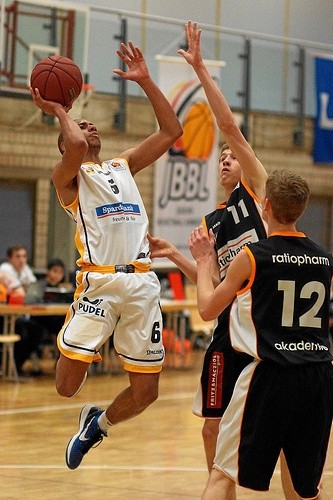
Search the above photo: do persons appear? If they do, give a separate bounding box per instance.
[188,171,333,500]
[147,19,268,500]
[0,243,42,376]
[25,258,74,379]
[27,40,183,469]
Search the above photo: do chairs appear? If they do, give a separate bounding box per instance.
[186,286,216,370]
[0,332,20,382]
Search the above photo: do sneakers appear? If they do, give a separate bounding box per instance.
[65,403,108,469]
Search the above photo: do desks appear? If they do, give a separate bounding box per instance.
[0,299,199,384]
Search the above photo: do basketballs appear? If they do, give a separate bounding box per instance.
[30,55,84,108]
